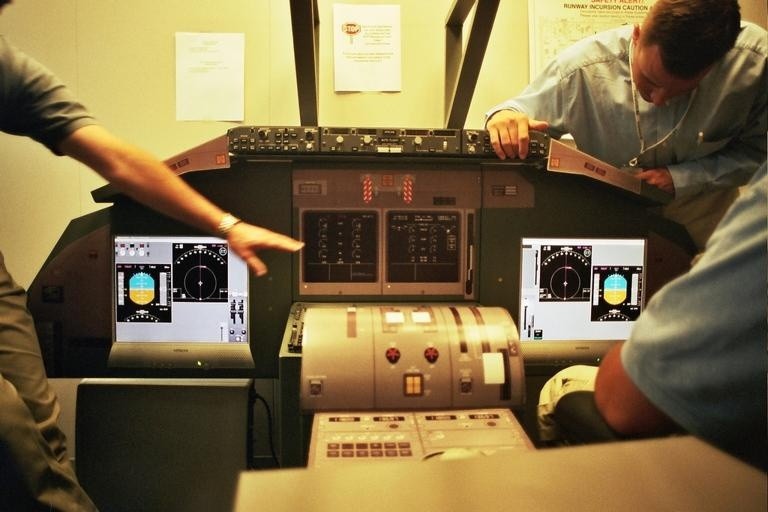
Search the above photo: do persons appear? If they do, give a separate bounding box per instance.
[532,158,766,485]
[0,0,308,512]
[480,0,766,248]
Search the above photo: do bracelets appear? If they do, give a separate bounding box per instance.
[215,214,240,239]
